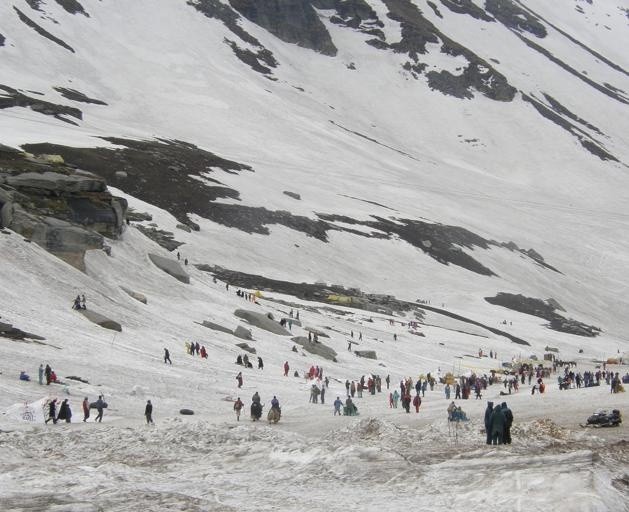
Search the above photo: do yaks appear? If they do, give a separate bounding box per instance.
[267,406,282,425]
[251,402,265,422]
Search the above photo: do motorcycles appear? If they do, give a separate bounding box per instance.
[578,408,623,432]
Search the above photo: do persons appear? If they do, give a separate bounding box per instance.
[176,245,629,446]
[20,370,30,381]
[145,400,153,424]
[81,294,88,308]
[164,348,172,365]
[38,362,108,425]
[75,295,81,310]
